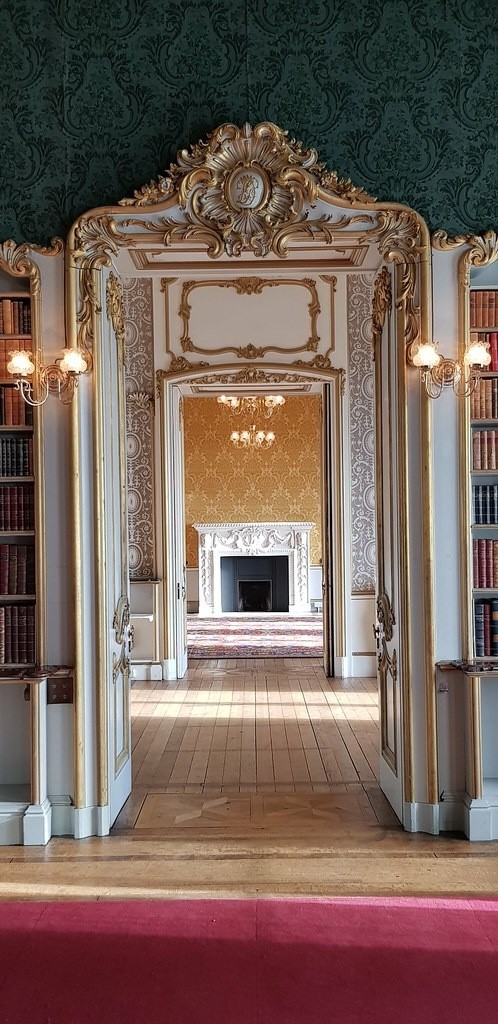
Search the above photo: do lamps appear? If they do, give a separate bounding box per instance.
[412,341,492,399]
[216,395,287,447]
[8,347,89,408]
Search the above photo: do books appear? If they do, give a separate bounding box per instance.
[466,292,497,659]
[2,297,36,670]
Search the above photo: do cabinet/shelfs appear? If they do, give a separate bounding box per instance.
[0,294,44,678]
[463,287,498,661]
